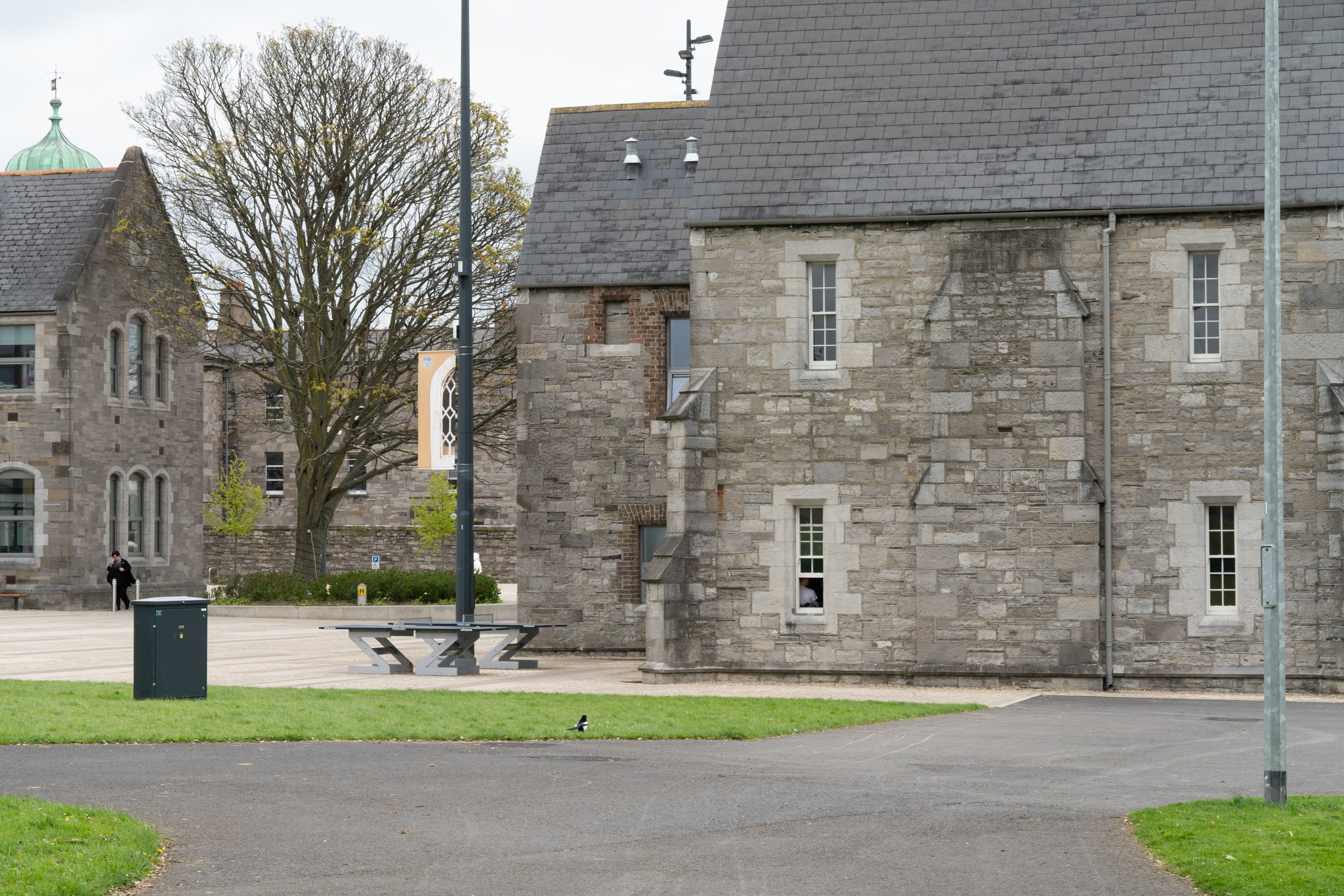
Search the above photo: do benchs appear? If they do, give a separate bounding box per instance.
[0,593,29,610]
[318,614,568,676]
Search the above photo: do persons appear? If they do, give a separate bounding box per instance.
[799,577,818,605]
[107,551,136,611]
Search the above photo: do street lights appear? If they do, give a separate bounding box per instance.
[664,18,714,100]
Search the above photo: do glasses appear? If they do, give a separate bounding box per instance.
[112,556,117,558]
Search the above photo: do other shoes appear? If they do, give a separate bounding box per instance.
[125,602,130,610]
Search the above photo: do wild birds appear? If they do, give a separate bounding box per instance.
[565,714,589,733]
